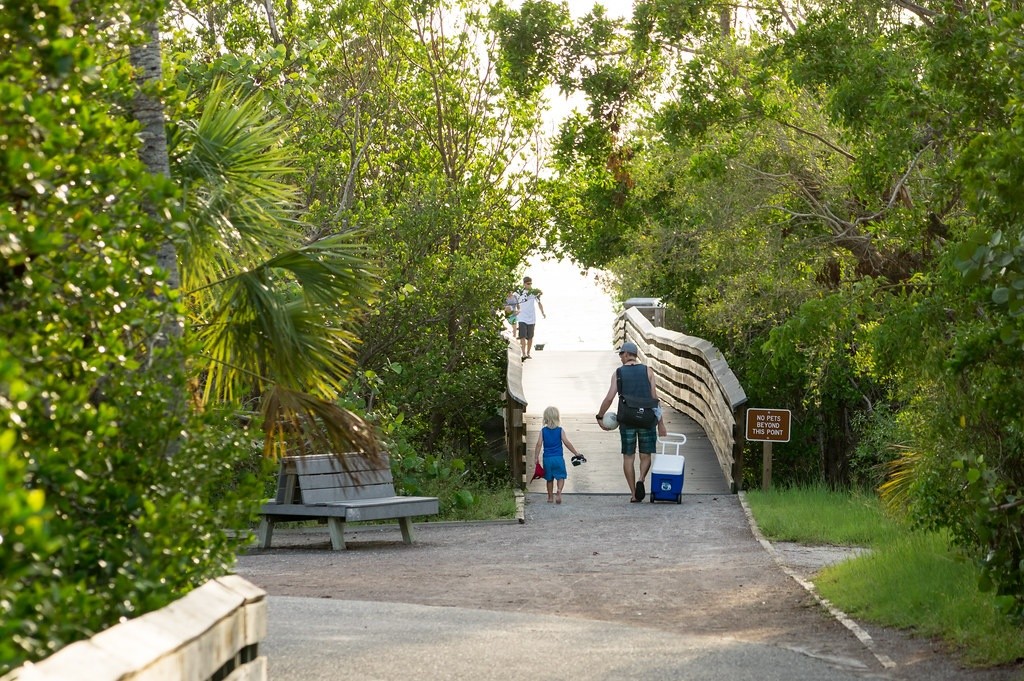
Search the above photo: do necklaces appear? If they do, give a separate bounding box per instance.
[626,359,636,362]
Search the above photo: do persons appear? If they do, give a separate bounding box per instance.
[534,406,580,503]
[506,292,521,337]
[597,342,667,502]
[518,277,546,361]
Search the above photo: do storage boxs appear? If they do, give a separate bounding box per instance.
[650,433,687,504]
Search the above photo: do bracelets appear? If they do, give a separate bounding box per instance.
[596,415,603,420]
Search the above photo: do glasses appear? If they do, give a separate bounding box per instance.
[526,282,532,285]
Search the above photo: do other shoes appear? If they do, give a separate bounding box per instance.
[571,454,587,466]
[522,355,528,362]
[527,355,532,359]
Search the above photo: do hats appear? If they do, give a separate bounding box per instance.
[529,463,546,484]
[615,342,637,355]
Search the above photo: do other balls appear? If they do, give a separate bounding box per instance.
[601,412,619,431]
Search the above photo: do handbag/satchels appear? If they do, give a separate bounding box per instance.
[616,394,662,428]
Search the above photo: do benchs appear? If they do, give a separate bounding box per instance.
[250,451,439,552]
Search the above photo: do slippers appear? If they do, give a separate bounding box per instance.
[630,497,641,502]
[634,481,645,501]
